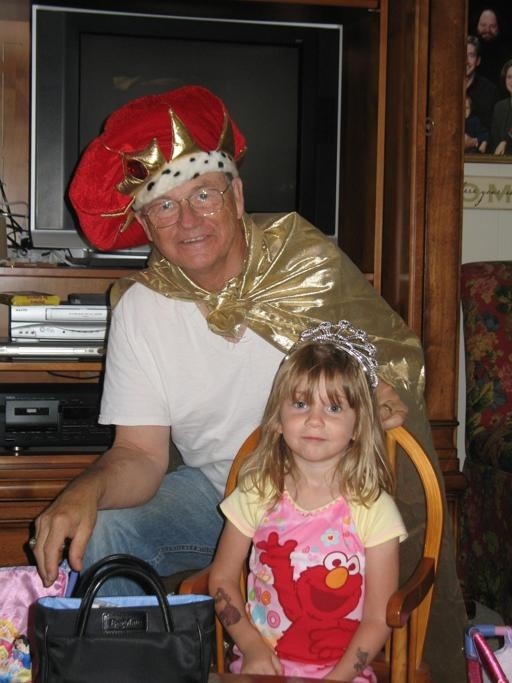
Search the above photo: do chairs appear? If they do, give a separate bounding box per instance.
[179,407,446,683]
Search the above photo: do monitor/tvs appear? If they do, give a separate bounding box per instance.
[30,3,344,266]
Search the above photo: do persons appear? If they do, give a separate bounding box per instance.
[464,9,512,158]
[33,83,470,682]
[207,320,409,683]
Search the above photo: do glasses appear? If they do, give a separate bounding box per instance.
[140,185,229,229]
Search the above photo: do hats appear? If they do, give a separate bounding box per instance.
[68,86,247,252]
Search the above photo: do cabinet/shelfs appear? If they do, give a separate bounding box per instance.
[0,263,147,566]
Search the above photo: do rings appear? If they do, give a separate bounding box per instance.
[384,403,392,415]
[29,539,35,547]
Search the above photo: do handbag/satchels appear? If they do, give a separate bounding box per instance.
[29,554,214,682]
